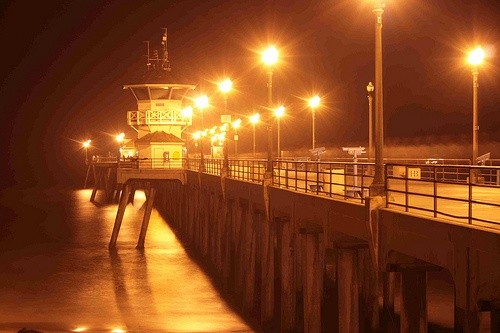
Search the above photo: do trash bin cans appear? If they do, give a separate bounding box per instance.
[322,168,345,199]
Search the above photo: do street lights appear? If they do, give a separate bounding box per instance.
[181,77,244,170]
[83,140,91,178]
[249,112,260,160]
[262,44,285,179]
[115,133,126,164]
[467,45,486,178]
[308,95,321,158]
[366,81,374,179]
